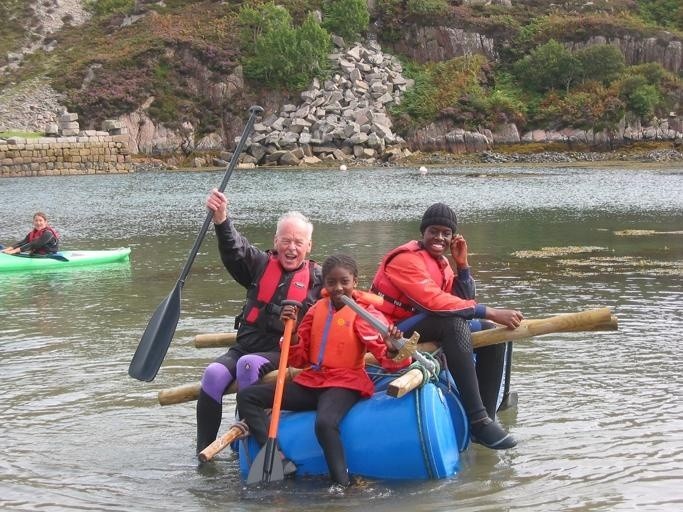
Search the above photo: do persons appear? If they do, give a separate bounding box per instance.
[369,202,524,450]
[235,252,413,495]
[195,190,326,463]
[2,211,59,258]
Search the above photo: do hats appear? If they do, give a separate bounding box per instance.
[421,203,457,232]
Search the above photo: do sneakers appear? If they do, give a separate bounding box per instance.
[470,415,518,450]
[282,458,297,475]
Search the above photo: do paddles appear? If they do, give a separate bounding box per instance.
[128,106,263,382]
[0,243,67,263]
[247,300,301,485]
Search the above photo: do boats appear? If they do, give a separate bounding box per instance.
[0,248,131,272]
